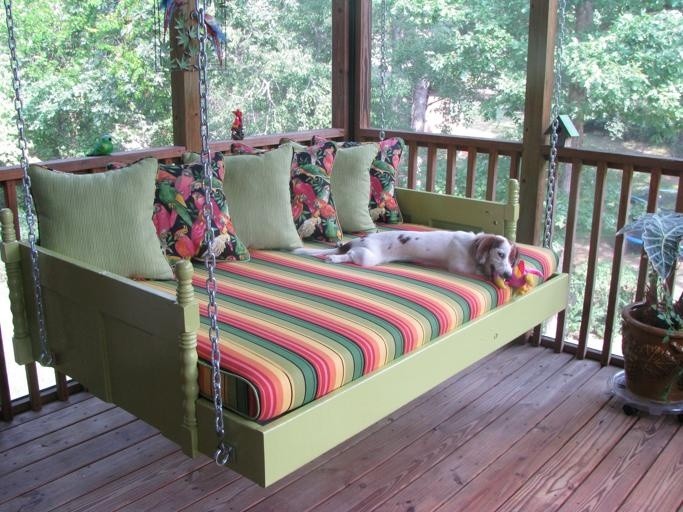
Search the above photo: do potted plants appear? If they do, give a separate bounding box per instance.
[614,209,683,403]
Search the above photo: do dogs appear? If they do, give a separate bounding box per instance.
[291,229,519,282]
